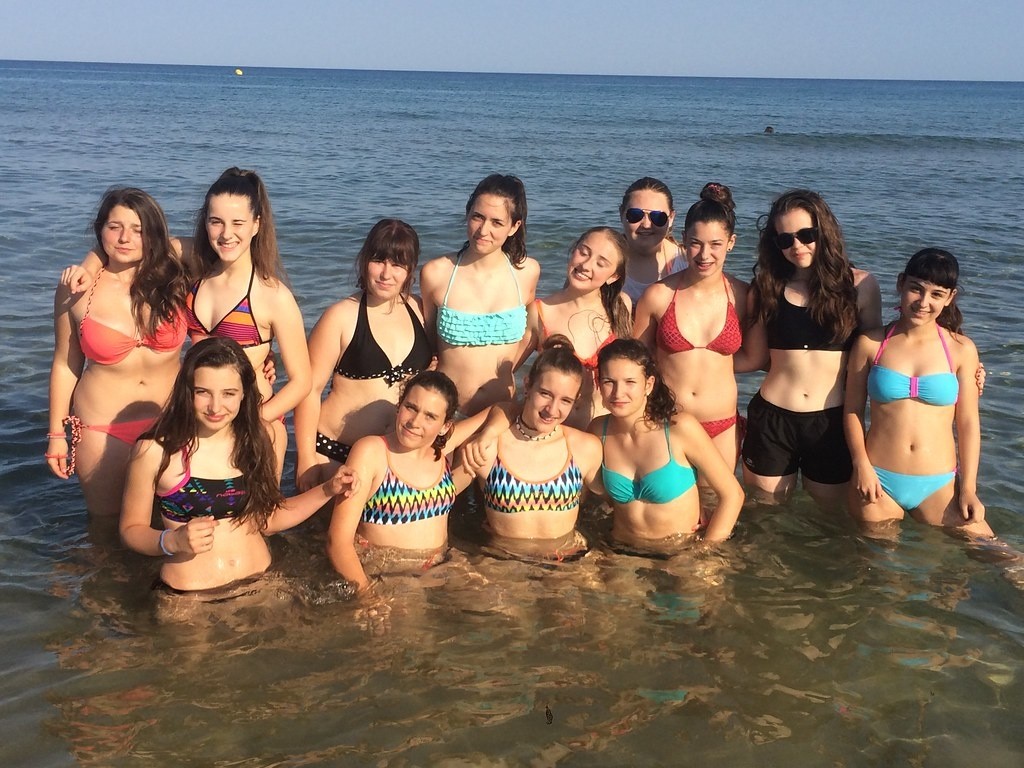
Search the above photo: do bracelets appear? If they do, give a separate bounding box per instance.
[160,529,176,555]
[44,431,69,458]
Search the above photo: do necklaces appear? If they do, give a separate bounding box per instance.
[515,415,561,442]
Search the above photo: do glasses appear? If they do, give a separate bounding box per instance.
[774,226,819,249]
[620,207,671,227]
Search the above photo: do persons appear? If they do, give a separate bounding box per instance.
[327,371,523,590]
[119,336,360,591]
[564,176,689,314]
[634,183,769,486]
[46,182,276,513]
[295,218,437,506]
[511,226,632,505]
[765,126,773,133]
[420,173,541,420]
[60,165,312,497]
[842,247,995,538]
[578,338,745,541]
[733,189,986,508]
[451,333,605,538]
